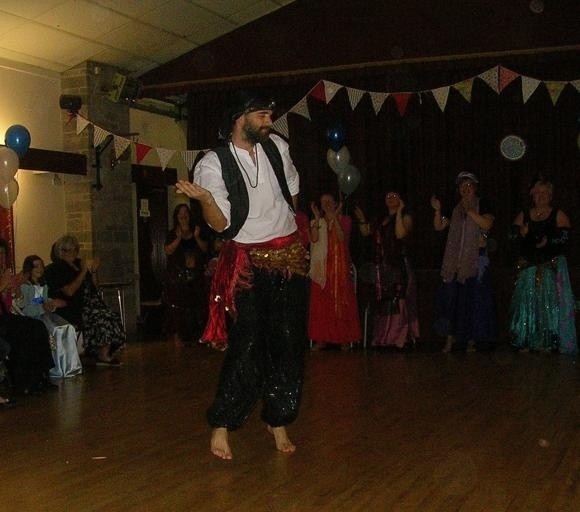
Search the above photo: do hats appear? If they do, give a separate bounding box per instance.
[223,89,276,123]
[458,172,480,184]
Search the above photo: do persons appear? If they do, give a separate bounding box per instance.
[0,392,20,411]
[12,253,87,379]
[41,234,129,367]
[175,88,310,462]
[0,238,60,399]
[176,200,226,353]
[293,172,578,357]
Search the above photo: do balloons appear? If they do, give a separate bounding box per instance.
[155,167,176,186]
[325,119,347,154]
[324,144,350,175]
[3,124,32,163]
[337,163,362,195]
[0,144,20,191]
[1,177,20,211]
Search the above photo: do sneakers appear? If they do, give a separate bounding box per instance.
[94,356,125,367]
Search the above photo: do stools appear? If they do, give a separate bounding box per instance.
[97,282,131,349]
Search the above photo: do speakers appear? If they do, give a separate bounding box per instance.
[59,94,81,111]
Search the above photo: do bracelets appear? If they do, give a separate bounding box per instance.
[90,271,98,274]
